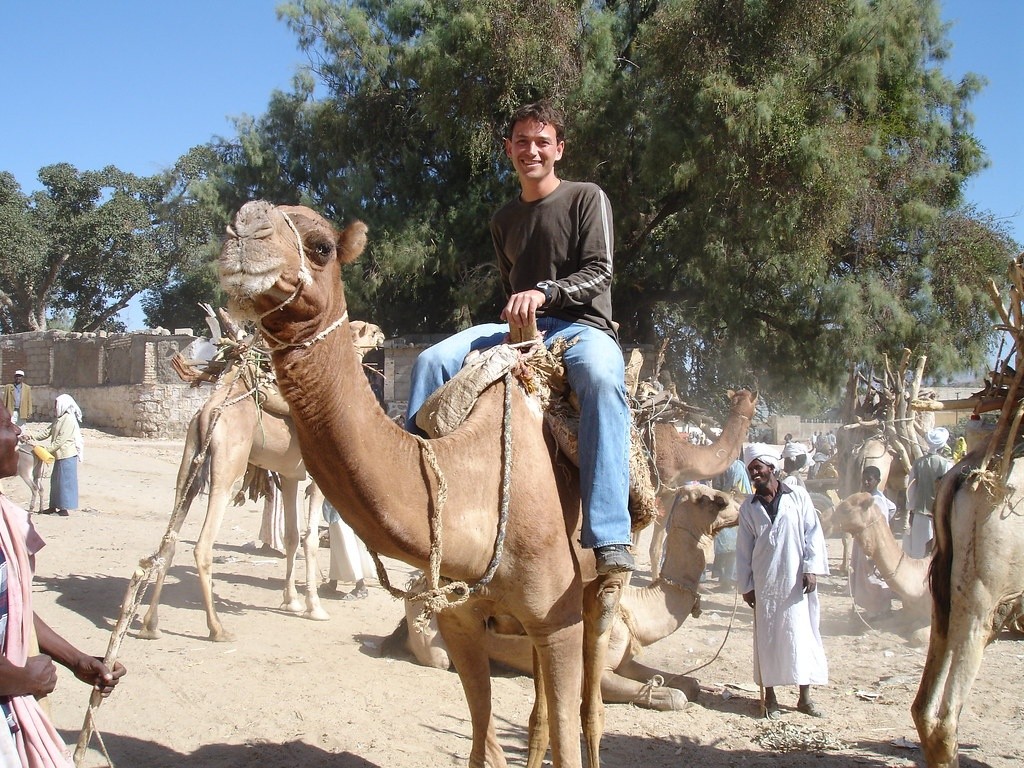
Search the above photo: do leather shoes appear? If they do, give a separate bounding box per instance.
[593,544,635,575]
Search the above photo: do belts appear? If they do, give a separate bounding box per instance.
[14,408,19,411]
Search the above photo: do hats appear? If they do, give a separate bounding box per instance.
[926,427,949,454]
[15,370,24,376]
[745,444,780,469]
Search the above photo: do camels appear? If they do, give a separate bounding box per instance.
[813,392,1024,768]
[216,197,760,768]
[134,318,386,638]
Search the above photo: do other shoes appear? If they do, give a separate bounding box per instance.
[320,583,335,591]
[797,700,821,717]
[43,509,56,514]
[59,510,68,516]
[342,589,369,600]
[765,697,780,720]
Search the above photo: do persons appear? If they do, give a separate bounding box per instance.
[806,430,837,502]
[902,427,949,559]
[710,459,753,589]
[0,399,127,768]
[257,470,305,556]
[3,369,32,430]
[735,442,832,721]
[779,434,793,461]
[844,465,897,620]
[406,105,636,577]
[952,436,968,466]
[318,496,370,603]
[679,430,724,448]
[781,442,806,489]
[19,393,84,516]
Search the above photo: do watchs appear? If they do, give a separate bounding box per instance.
[533,281,553,308]
